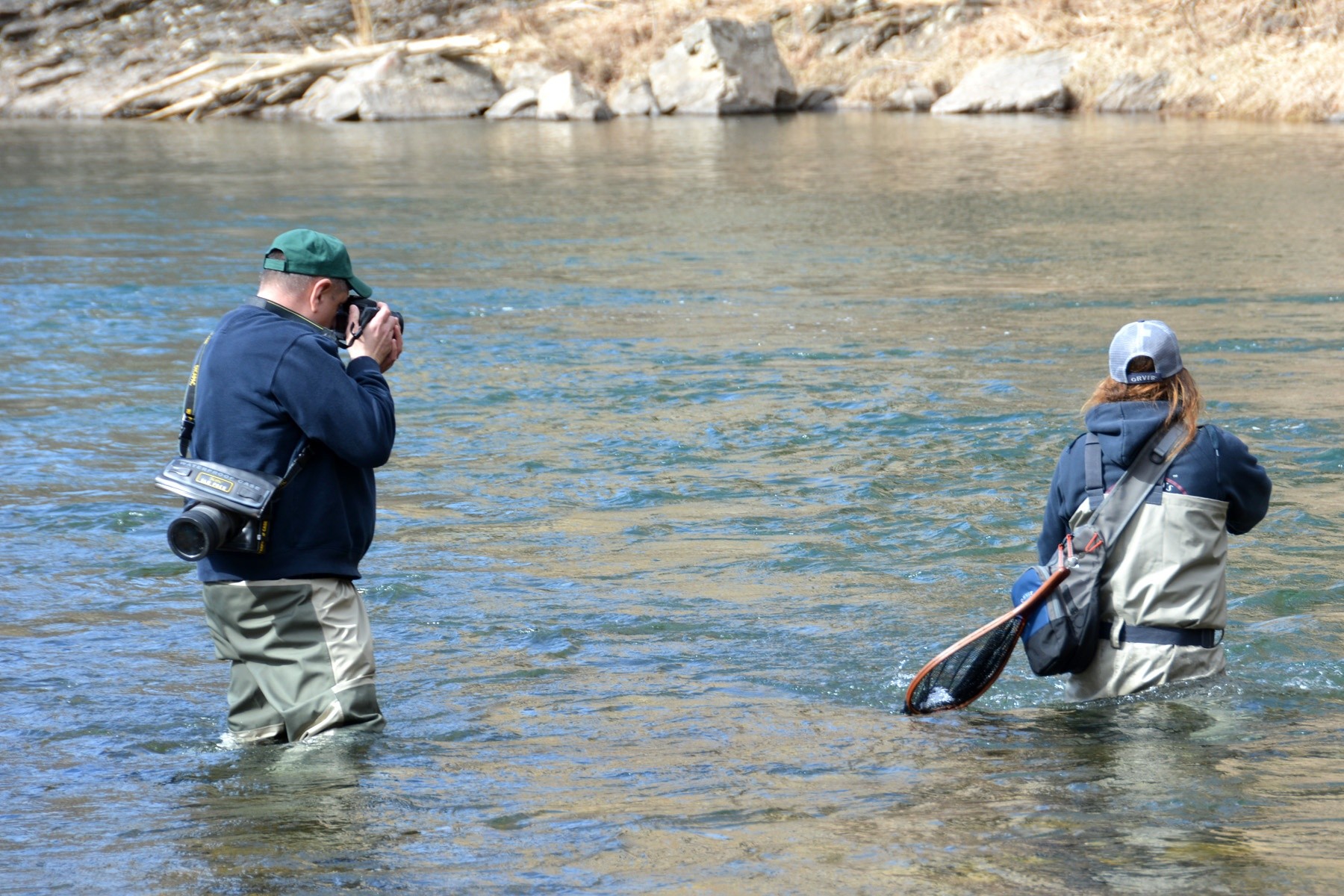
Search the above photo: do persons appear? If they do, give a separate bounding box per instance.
[177,229,403,744]
[1037,321,1272,702]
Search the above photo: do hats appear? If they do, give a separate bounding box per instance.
[263,229,373,298]
[1108,319,1184,384]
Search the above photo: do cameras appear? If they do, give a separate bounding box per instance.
[334,293,405,339]
[167,501,232,562]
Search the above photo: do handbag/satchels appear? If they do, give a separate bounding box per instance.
[1011,526,1107,678]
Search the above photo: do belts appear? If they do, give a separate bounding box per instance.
[1098,620,1215,650]
